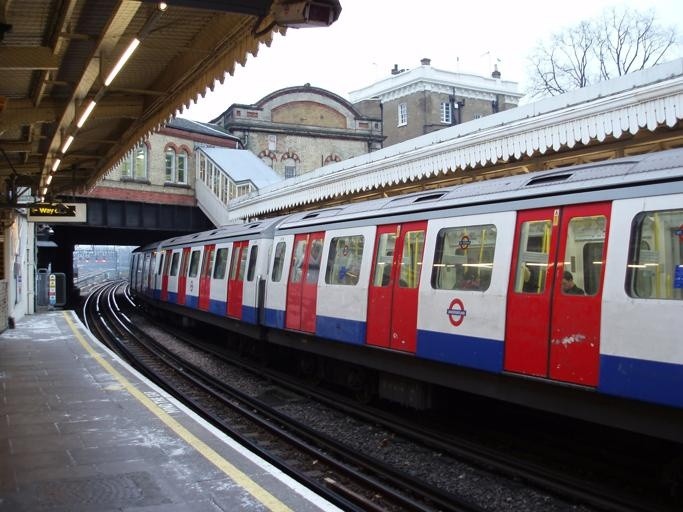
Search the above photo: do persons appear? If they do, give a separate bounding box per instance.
[563,270,586,295]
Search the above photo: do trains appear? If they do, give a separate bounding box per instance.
[127,145,682,446]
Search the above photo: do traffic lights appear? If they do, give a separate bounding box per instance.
[101,256,105,263]
[86,257,88,263]
[95,257,98,263]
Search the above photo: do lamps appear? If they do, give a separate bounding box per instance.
[36,3,170,202]
